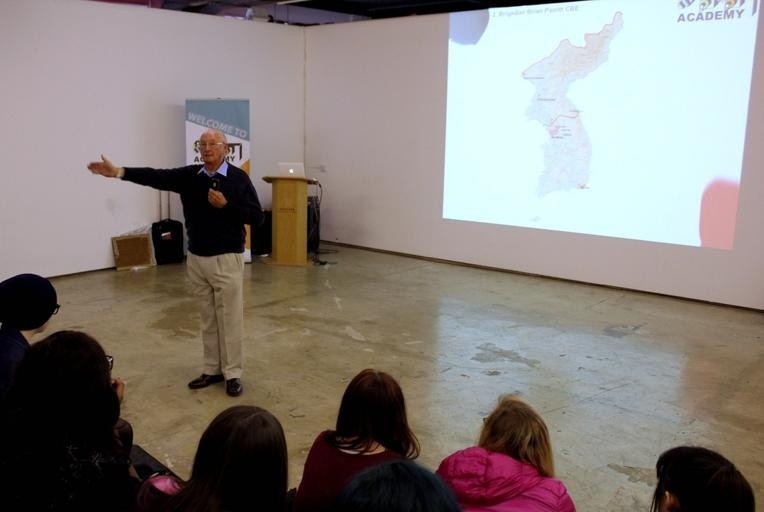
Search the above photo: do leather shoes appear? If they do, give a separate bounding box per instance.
[226,378,243,397]
[188,373,223,389]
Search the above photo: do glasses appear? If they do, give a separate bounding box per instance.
[52,304,60,315]
[106,355,114,371]
[197,143,223,150]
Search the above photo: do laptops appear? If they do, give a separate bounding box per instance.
[278,162,306,177]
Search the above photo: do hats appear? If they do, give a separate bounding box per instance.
[1,274,57,330]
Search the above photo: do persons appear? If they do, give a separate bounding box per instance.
[134,404,297,511]
[1,330,156,511]
[294,366,420,512]
[648,444,756,512]
[87,127,263,398]
[323,458,463,512]
[434,390,579,512]
[1,271,62,388]
